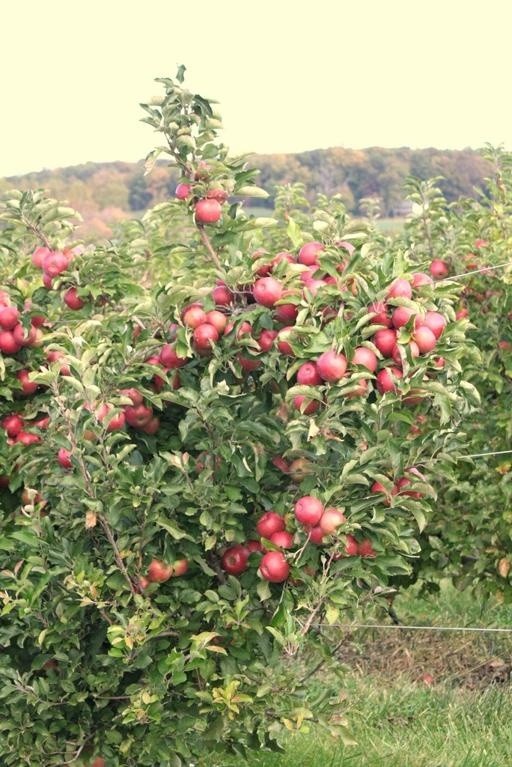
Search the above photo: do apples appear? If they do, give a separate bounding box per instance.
[0,160,512,592]
